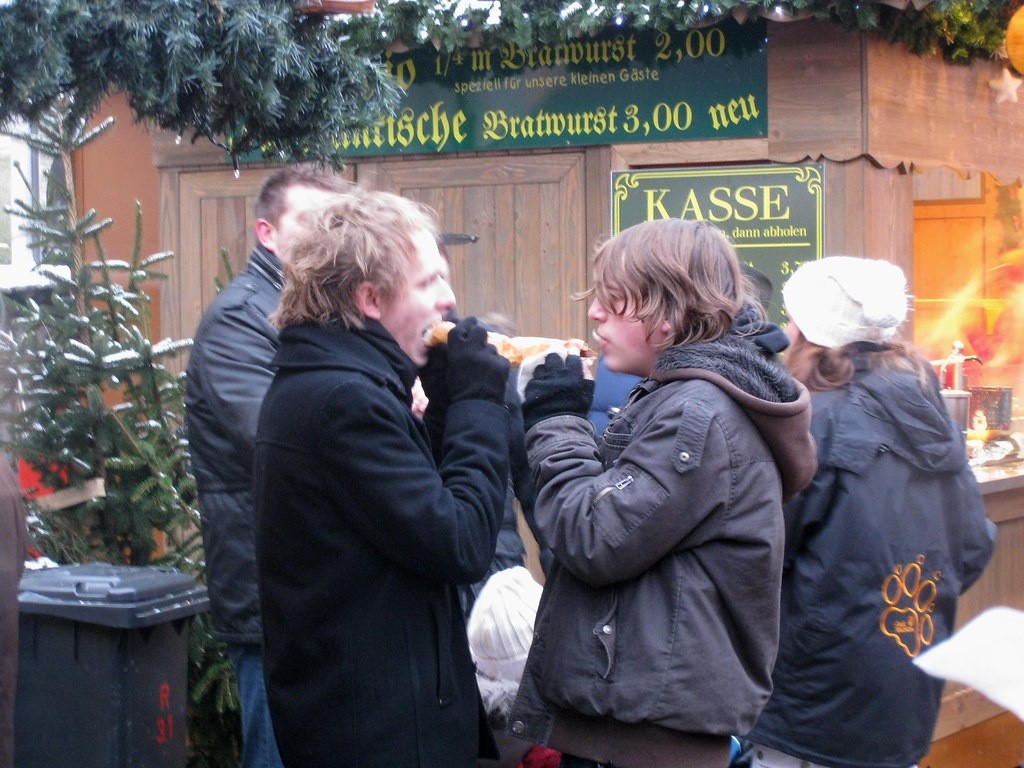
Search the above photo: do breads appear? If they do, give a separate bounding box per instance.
[427,322,523,367]
[511,337,593,361]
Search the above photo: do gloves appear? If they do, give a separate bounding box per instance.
[416,315,510,411]
[519,352,597,429]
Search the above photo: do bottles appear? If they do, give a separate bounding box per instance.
[940,386,1012,460]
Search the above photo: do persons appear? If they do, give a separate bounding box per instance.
[254,189,774,768]
[186,162,361,768]
[742,257,997,768]
[506,219,818,768]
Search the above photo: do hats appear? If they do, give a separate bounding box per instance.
[784,255,909,349]
[465,565,545,681]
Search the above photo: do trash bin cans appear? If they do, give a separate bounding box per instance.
[13,557,214,768]
